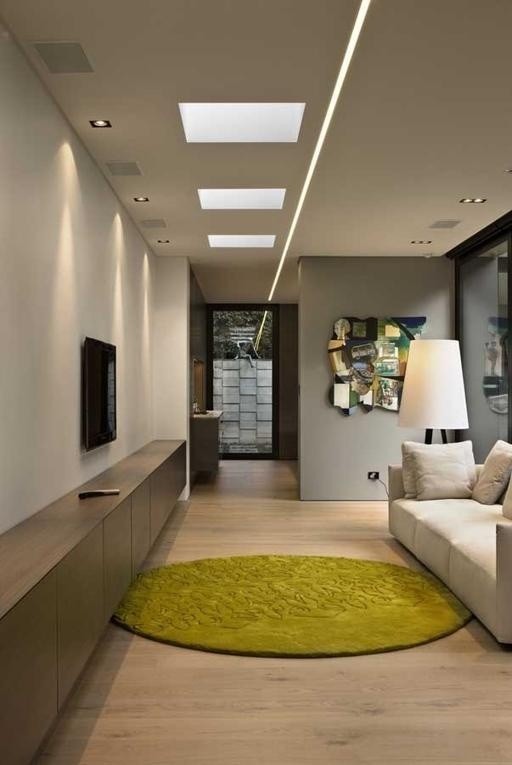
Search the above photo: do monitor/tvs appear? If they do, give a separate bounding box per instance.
[82,336,116,451]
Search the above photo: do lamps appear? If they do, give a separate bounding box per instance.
[395,338,470,444]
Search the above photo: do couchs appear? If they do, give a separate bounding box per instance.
[387,461,512,650]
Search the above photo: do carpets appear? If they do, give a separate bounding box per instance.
[111,556,474,660]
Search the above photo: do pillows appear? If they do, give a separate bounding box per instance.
[401,438,512,519]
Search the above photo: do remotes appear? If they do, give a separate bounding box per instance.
[79,489,120,499]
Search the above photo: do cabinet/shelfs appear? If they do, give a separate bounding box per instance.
[2,437,187,764]
